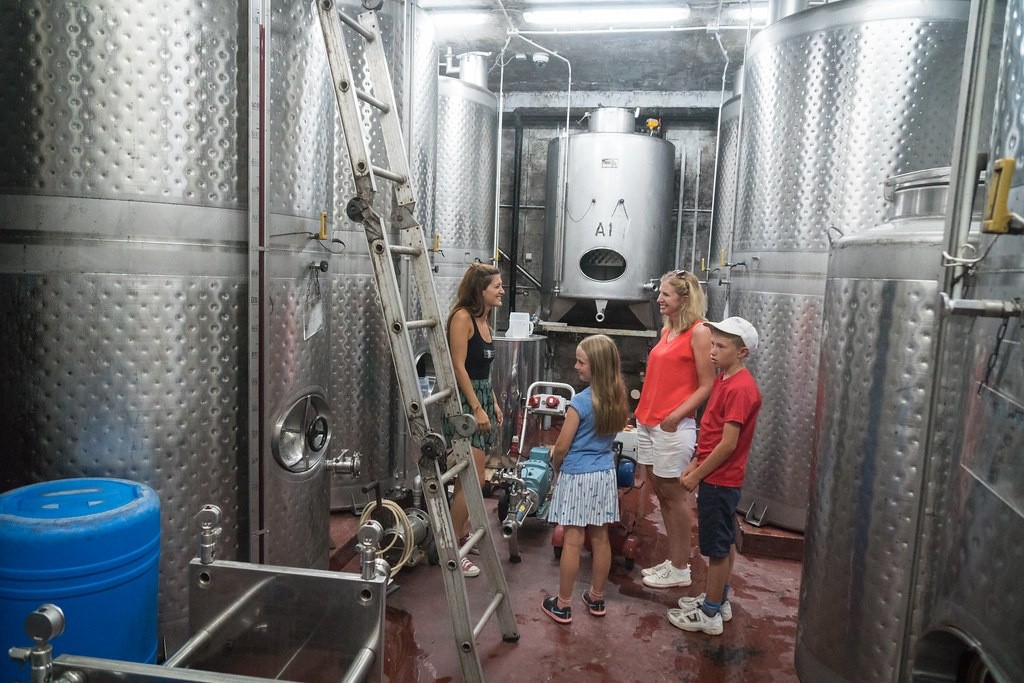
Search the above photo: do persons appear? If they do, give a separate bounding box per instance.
[633,269,719,589]
[667,317,762,638]
[541,333,629,623]
[437,264,505,578]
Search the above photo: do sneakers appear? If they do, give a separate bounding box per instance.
[667,609,723,635]
[439,557,480,576]
[541,597,572,623]
[643,563,692,587]
[679,592,732,621]
[642,559,669,576]
[460,534,480,554]
[582,591,606,614]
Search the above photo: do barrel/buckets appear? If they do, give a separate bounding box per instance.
[0,476,158,683]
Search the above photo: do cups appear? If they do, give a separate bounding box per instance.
[510,312,533,338]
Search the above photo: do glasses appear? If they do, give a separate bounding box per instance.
[671,269,689,290]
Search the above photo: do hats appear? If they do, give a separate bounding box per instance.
[702,316,759,354]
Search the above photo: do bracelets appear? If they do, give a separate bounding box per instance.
[473,404,482,414]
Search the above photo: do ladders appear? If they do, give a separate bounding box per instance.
[316,0,520,683]
